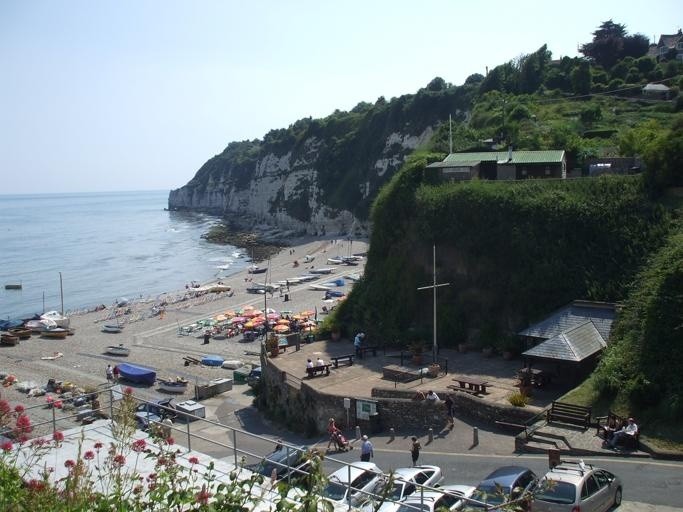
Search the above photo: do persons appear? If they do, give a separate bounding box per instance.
[314,357,324,375]
[412,389,440,400]
[161,415,172,437]
[354,334,361,353]
[601,414,638,451]
[409,437,421,466]
[305,359,313,368]
[445,394,454,427]
[327,418,348,453]
[276,439,282,450]
[106,364,119,386]
[360,435,373,462]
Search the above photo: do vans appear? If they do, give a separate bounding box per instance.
[260,444,311,480]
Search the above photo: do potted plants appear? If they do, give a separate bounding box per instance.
[472,319,496,357]
[496,336,512,360]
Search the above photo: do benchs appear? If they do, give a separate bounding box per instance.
[446,384,492,395]
[547,399,592,430]
[596,409,640,450]
[305,363,332,377]
[331,354,355,368]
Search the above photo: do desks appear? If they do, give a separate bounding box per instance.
[518,368,544,386]
[382,363,421,382]
[452,376,488,394]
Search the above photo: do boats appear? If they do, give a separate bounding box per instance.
[5,279,23,289]
[246,236,368,297]
[158,382,189,393]
[1,320,71,346]
[186,277,232,296]
[102,319,125,333]
[103,343,130,357]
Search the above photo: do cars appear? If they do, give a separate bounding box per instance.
[137,398,179,423]
[132,411,161,430]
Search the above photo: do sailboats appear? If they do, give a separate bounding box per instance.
[40,272,70,328]
